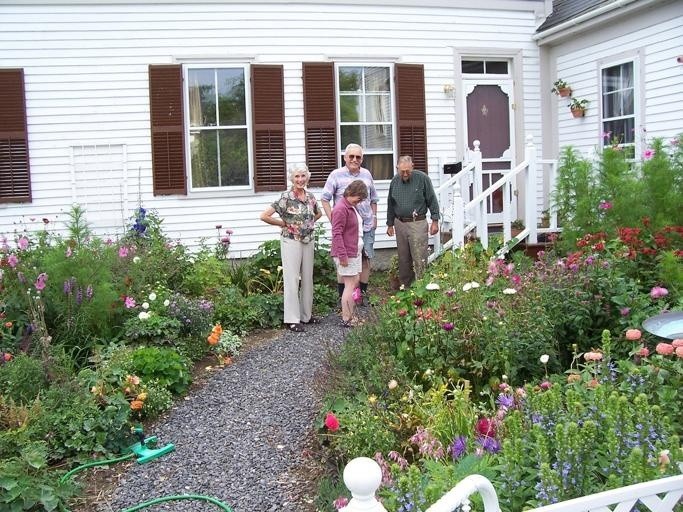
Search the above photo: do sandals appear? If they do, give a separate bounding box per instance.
[340,315,366,327]
[300,315,320,324]
[288,322,305,332]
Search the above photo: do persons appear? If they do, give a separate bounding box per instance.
[319,144,379,306]
[386,155,440,286]
[330,179,368,326]
[261,163,322,331]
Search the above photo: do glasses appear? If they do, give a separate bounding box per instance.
[349,154,361,159]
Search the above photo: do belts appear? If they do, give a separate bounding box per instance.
[396,215,426,222]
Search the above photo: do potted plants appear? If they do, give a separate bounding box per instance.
[540,207,550,228]
[511,218,524,238]
[551,79,571,96]
[567,98,589,118]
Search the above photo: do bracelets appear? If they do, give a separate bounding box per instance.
[372,215,377,218]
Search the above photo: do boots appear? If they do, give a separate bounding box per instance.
[359,281,370,307]
[337,282,345,308]
